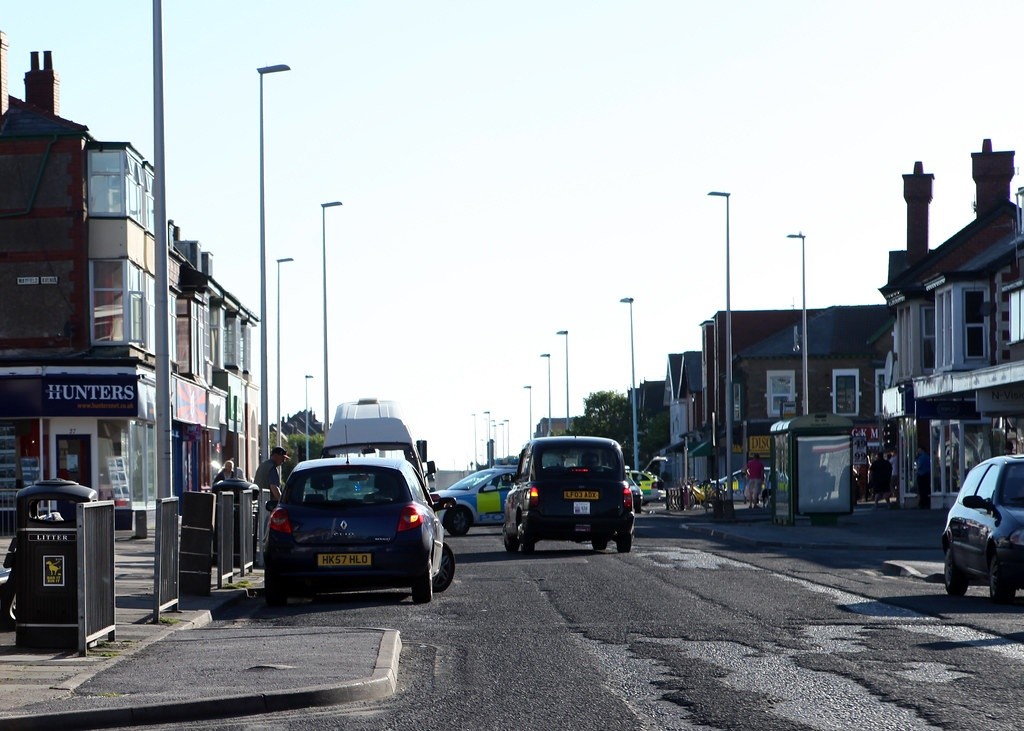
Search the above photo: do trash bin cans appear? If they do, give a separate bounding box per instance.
[14,478,99,653]
[211,478,260,569]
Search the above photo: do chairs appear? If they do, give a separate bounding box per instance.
[580,452,599,467]
[374,490,393,502]
[302,493,327,505]
[310,472,337,506]
[363,472,400,501]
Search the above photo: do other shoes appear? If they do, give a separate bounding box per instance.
[749,505,762,509]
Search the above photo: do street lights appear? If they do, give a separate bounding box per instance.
[621,297,641,474]
[276,257,295,522]
[306,374,316,462]
[473,409,510,463]
[321,201,345,460]
[556,331,571,435]
[541,353,551,436]
[787,232,810,418]
[706,190,736,526]
[256,62,291,556]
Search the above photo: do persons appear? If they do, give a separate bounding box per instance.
[213,457,246,481]
[740,454,771,509]
[853,447,930,510]
[253,446,291,502]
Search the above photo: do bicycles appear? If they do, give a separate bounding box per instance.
[670,478,724,509]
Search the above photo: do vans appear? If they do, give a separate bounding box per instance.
[319,397,426,508]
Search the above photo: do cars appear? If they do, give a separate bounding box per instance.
[941,455,1024,604]
[503,435,636,556]
[623,470,666,504]
[425,464,531,536]
[262,454,457,603]
[704,467,777,503]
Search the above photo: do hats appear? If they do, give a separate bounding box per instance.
[272,445,290,459]
